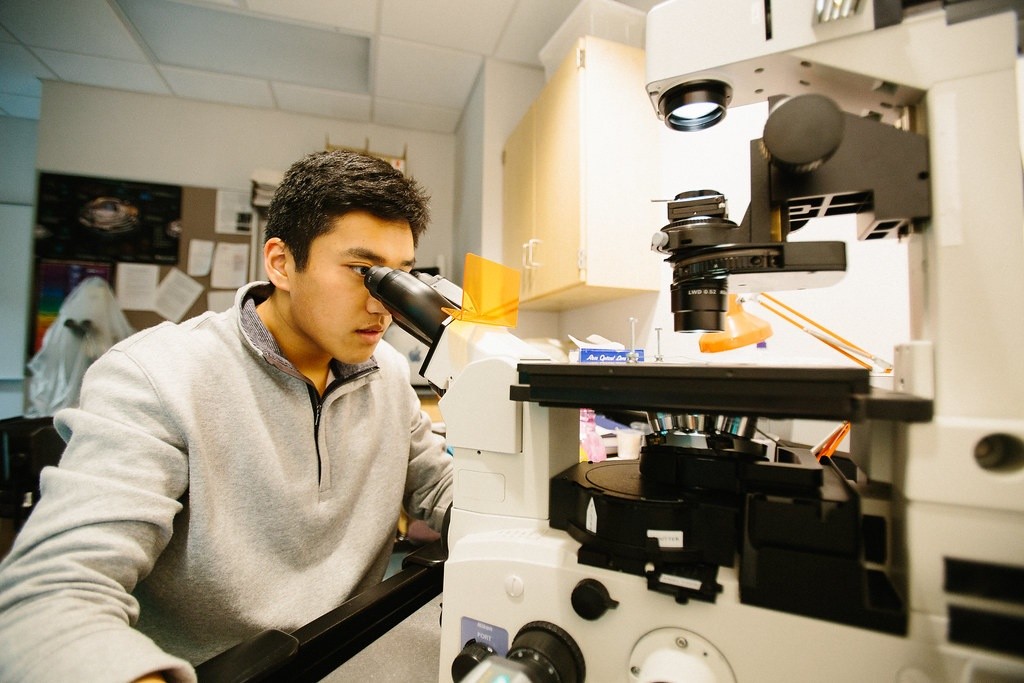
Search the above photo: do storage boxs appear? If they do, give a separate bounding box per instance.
[540,0,646,82]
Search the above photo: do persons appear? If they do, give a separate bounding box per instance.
[0,152,447,683]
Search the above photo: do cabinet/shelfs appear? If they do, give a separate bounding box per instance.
[500,35,663,313]
